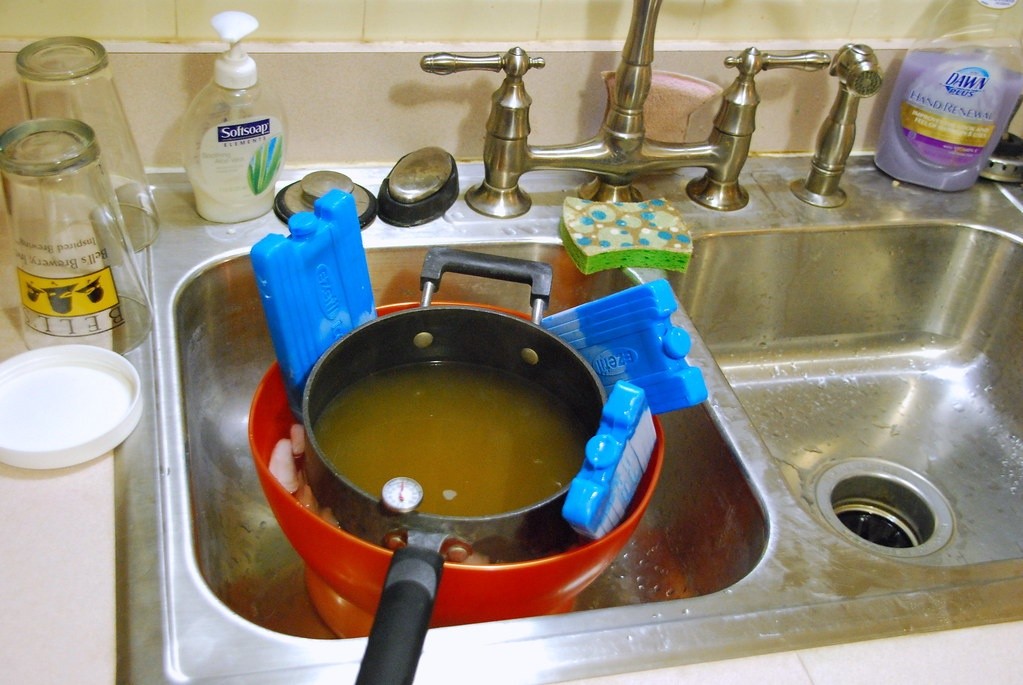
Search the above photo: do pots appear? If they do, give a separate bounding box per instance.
[303,246,554,685]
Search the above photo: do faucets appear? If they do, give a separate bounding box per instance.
[602,0,663,143]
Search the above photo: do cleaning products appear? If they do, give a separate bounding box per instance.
[182,9,288,225]
[874,0,1023,194]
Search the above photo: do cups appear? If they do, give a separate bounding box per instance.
[0,118,153,358]
[14,37,157,253]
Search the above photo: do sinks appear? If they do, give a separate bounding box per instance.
[152,233,773,685]
[658,221,1023,653]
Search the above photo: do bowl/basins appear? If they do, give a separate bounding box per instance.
[249,301,665,639]
